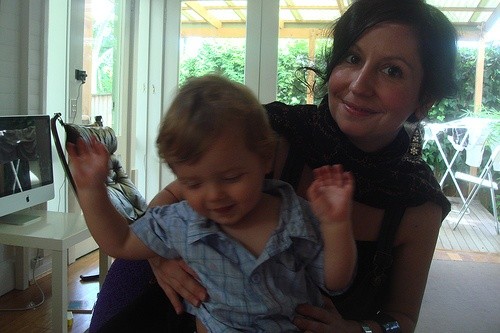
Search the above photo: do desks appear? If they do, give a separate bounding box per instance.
[0,206,112,333]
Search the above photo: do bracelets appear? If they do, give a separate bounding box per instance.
[373,311,401,333]
[359,320,375,333]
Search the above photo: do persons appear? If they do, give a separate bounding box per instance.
[82,0,459,333]
[67,76,356,333]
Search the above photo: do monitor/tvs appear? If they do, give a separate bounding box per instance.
[0,115,55,225]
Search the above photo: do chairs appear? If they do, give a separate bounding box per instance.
[62,119,147,226]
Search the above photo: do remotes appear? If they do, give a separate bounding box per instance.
[79,274,99,281]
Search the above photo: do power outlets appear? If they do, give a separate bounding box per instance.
[32,257,44,268]
